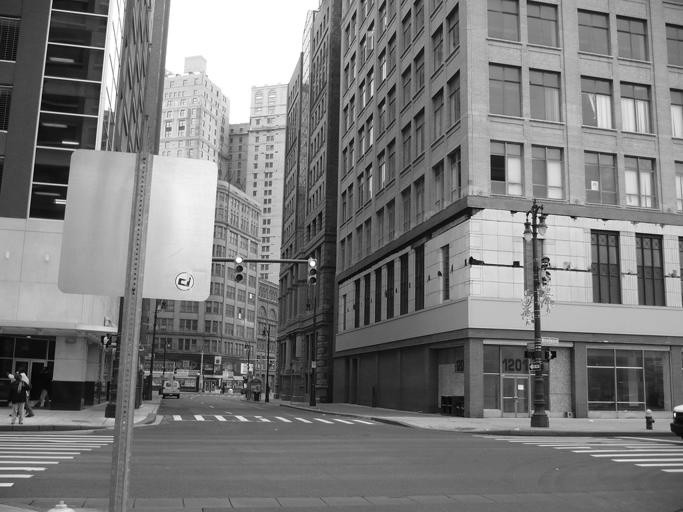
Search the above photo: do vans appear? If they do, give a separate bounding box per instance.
[162,380,181,399]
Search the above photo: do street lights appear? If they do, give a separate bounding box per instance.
[523,197,552,427]
[261,324,271,403]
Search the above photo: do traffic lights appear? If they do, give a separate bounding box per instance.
[307,257,318,286]
[233,255,245,283]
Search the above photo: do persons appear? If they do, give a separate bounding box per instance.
[16,366,34,417]
[7,374,30,425]
[6,369,19,416]
[38,366,51,409]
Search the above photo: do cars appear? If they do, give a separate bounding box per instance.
[669,404,682,441]
